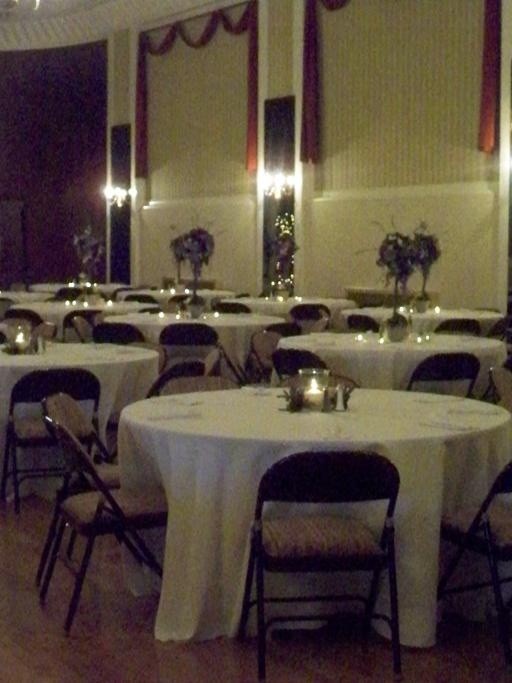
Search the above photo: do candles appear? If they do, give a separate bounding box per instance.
[305,378,324,405]
[13,332,26,353]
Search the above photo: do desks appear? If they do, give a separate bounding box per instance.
[116,289,237,310]
[119,387,511,647]
[221,296,357,328]
[15,302,159,342]
[0,342,159,461]
[103,314,285,394]
[30,283,133,300]
[340,306,504,332]
[272,331,507,400]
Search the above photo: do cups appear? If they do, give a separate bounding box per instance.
[297,367,331,408]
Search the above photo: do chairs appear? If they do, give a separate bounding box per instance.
[265,322,301,336]
[237,450,400,682]
[2,368,100,514]
[63,309,103,342]
[214,303,250,314]
[125,295,157,304]
[160,375,239,397]
[434,318,479,337]
[147,361,207,398]
[91,313,106,323]
[159,322,243,383]
[251,331,283,382]
[290,304,324,332]
[37,411,167,633]
[4,308,56,338]
[35,391,122,588]
[317,305,329,320]
[437,458,512,638]
[407,352,480,397]
[138,307,162,313]
[486,317,509,341]
[71,314,93,342]
[271,347,327,382]
[168,294,206,307]
[55,288,84,300]
[347,315,380,332]
[279,373,356,389]
[92,322,144,343]
[0,298,15,322]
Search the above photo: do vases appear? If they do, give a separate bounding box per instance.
[187,305,204,317]
[386,324,407,343]
[416,300,431,313]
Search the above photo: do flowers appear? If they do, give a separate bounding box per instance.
[414,228,442,300]
[182,225,215,304]
[170,234,189,284]
[376,231,418,324]
[72,225,103,282]
[269,234,299,291]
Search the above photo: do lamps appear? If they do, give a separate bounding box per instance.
[103,186,138,208]
[258,172,294,199]
